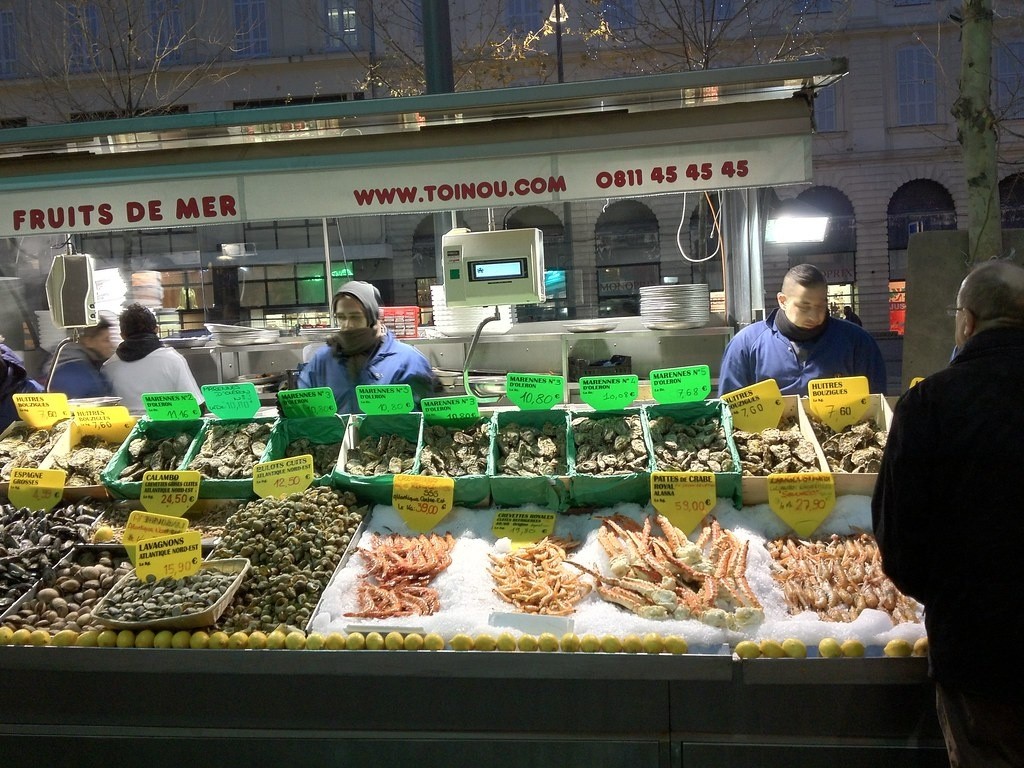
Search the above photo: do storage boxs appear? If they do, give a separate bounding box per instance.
[0,391,896,632]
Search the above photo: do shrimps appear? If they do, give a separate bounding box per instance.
[765,524,921,625]
[487,532,592,616]
[342,525,454,618]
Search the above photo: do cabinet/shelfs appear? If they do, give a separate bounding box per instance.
[175,325,735,416]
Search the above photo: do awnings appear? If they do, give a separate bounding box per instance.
[0,56,851,163]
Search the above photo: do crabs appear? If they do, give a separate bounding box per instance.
[562,512,765,630]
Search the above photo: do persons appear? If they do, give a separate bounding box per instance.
[297,280,447,413]
[98,303,206,417]
[843,306,862,328]
[717,264,887,400]
[41,317,115,403]
[871,259,1024,768]
[0,335,46,435]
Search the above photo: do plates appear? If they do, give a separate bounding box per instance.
[638,284,709,329]
[563,322,619,333]
[36,311,67,350]
[228,370,284,383]
[98,307,123,348]
[160,338,207,348]
[299,328,342,340]
[204,322,280,346]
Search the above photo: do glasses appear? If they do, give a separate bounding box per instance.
[944,303,976,320]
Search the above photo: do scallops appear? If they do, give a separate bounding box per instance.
[0,502,134,634]
[94,567,238,622]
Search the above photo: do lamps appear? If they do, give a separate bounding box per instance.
[762,197,830,244]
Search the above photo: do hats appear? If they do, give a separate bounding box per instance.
[336,280,381,328]
